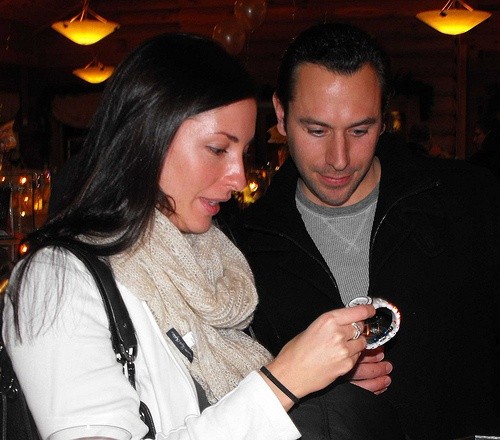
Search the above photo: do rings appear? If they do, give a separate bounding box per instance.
[352,321,361,340]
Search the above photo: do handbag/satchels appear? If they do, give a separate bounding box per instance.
[1,237,157,440]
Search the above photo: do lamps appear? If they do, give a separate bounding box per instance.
[51,0,120,45]
[415,0,492,35]
[72,53,116,84]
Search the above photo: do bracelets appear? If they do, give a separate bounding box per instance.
[259,366,302,405]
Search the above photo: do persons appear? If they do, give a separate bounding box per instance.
[225,24,499,440]
[1,32,376,440]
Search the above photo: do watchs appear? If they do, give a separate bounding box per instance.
[345,295,402,350]
